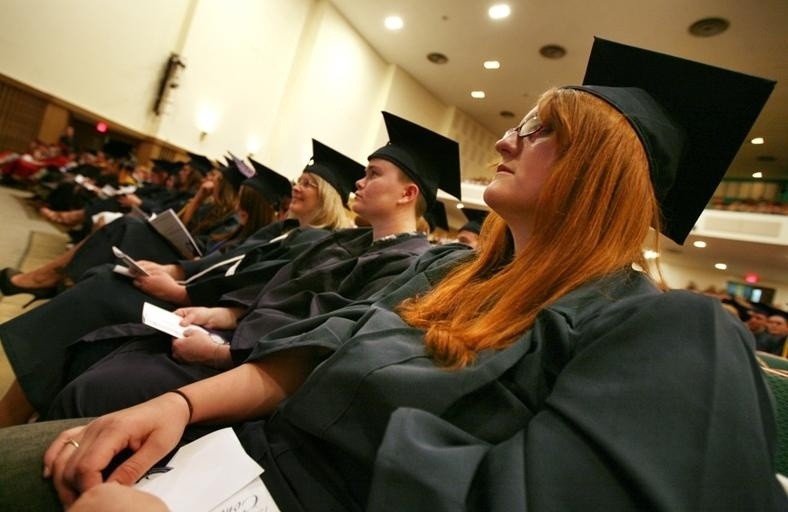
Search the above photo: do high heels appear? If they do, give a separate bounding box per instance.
[0,267,64,308]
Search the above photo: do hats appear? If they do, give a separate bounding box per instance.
[302,138,367,203]
[151,151,291,212]
[368,110,461,211]
[558,36,777,246]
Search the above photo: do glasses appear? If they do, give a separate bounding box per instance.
[503,113,556,139]
[293,176,318,189]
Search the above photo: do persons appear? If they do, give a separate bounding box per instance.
[0,37,787,511]
